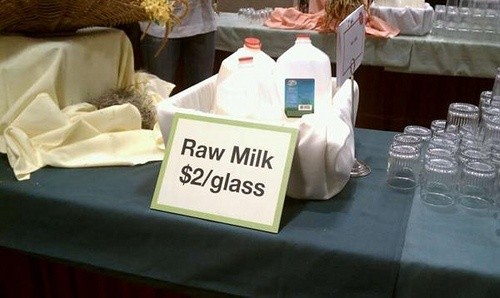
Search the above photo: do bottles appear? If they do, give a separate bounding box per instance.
[211,37,277,125]
[274,30,333,127]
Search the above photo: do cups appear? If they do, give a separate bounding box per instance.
[429,3,500,43]
[237,6,272,26]
[383,64,499,208]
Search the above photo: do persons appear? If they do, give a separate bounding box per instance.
[136,0,220,92]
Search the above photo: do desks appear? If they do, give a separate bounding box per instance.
[0,127,500,298]
[214,12,500,134]
